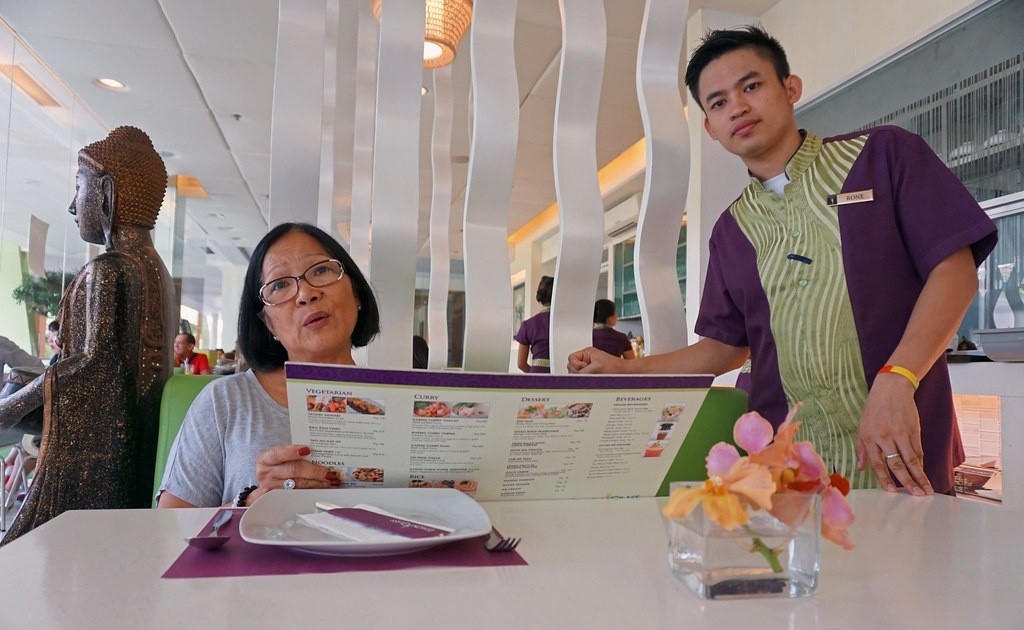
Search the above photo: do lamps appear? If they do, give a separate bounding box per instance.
[369,0,473,68]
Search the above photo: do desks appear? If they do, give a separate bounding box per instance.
[0,485,1024,630]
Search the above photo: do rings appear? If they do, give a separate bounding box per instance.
[884,453,900,459]
[283,478,296,489]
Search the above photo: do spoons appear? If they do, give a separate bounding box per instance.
[184,510,233,551]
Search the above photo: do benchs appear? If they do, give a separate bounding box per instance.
[152,373,749,507]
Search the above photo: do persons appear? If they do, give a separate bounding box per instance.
[413,335,428,369]
[174,332,210,375]
[593,299,635,359]
[1,320,60,509]
[567,26,998,495]
[516,276,554,374]
[213,342,250,375]
[155,223,380,508]
[1,126,180,548]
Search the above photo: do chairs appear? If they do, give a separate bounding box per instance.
[0,424,44,532]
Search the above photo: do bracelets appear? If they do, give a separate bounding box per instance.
[877,366,919,391]
[232,485,257,507]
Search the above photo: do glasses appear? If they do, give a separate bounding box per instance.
[255,254,344,306]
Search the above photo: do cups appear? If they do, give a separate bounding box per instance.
[185,364,194,375]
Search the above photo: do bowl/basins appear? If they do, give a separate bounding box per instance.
[973,327,1024,362]
[644,423,673,457]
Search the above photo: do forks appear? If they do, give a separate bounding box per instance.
[482,528,521,553]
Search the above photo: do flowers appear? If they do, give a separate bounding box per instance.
[659,402,859,573]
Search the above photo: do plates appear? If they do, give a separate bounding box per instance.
[306,395,591,418]
[239,489,492,557]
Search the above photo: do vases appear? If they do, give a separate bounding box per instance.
[973,327,1024,362]
[669,481,822,601]
